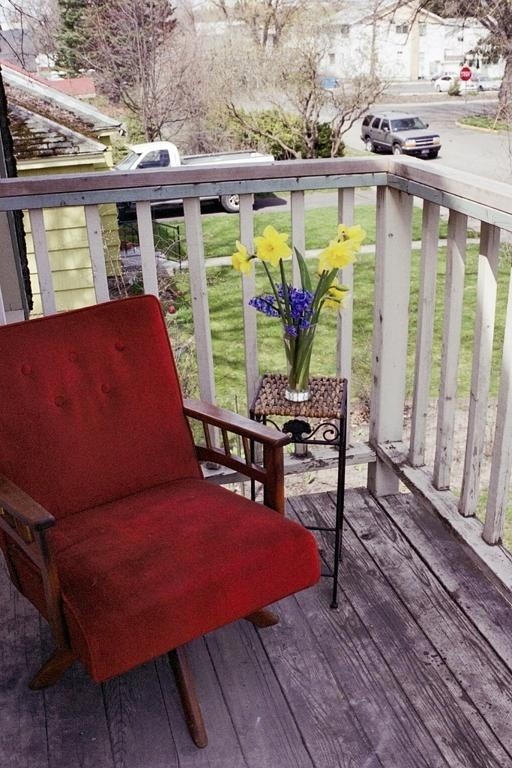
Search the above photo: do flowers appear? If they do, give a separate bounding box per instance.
[230,221,367,392]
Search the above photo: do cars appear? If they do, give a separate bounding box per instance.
[434,75,501,92]
[360,110,442,158]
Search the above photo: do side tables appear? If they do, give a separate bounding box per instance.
[250,373,348,608]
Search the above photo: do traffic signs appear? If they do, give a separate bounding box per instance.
[460,67,472,81]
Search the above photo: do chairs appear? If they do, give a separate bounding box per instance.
[0,294,322,750]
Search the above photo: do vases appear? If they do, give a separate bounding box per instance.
[280,323,318,402]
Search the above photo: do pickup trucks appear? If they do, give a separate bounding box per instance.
[115,141,276,212]
